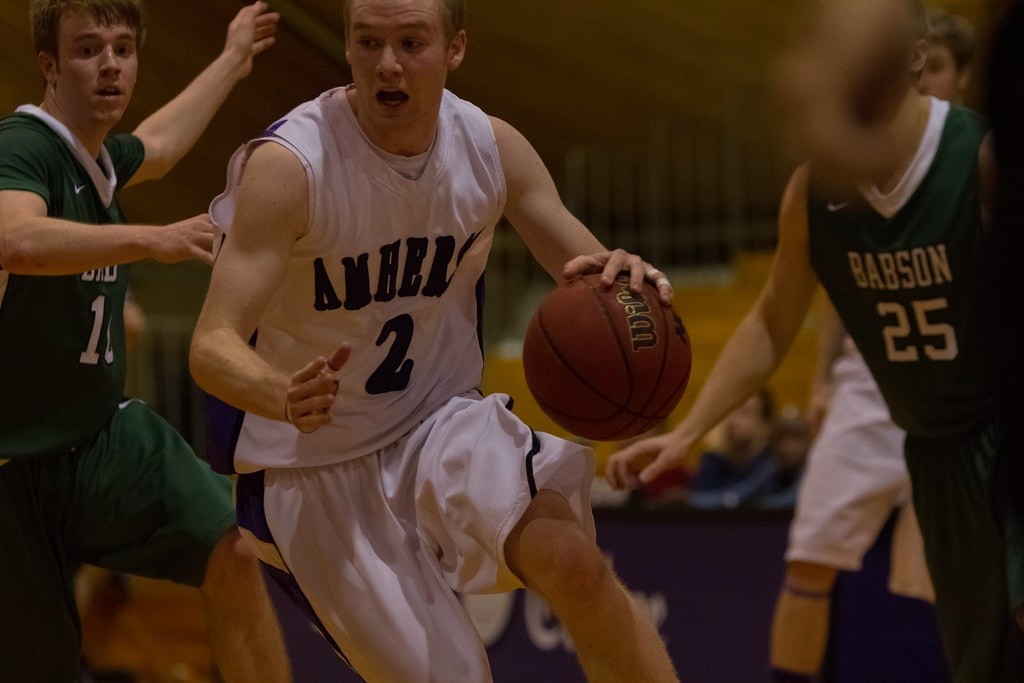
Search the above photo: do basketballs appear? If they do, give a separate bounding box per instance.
[523,272,693,443]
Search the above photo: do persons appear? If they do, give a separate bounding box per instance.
[191,0,673,683]
[606,0,1024,683]
[0,0,293,683]
